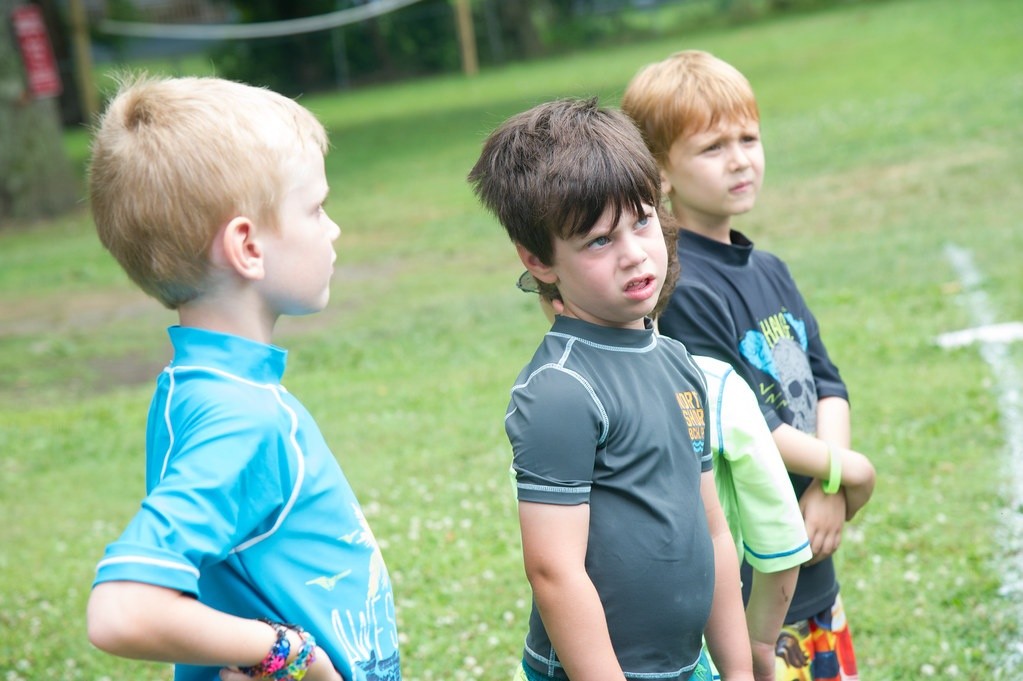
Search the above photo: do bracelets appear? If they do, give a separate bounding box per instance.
[821,443,846,495]
[240,618,290,679]
[270,622,315,680]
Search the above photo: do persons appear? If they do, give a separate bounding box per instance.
[475,100,754,681]
[618,46,876,680]
[515,205,812,681]
[81,71,403,680]
